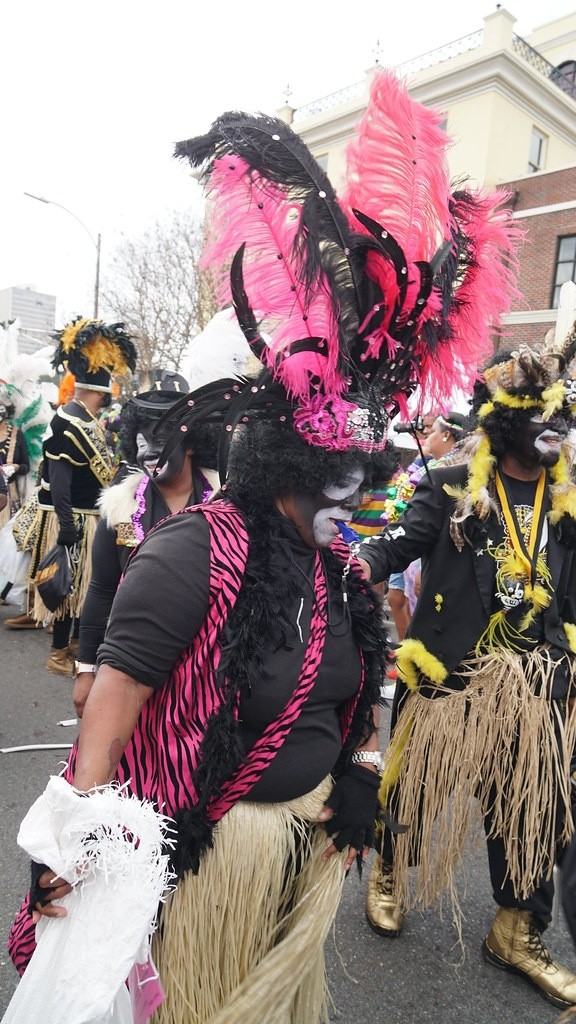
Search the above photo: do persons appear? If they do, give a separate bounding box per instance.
[357,340,576,1008]
[334,405,481,681]
[29,382,375,1024]
[71,391,222,722]
[1,355,143,678]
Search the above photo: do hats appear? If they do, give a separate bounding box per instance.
[120,370,191,428]
[48,374,120,411]
[50,315,137,393]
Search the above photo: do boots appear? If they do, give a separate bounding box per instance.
[3,590,44,629]
[482,904,576,1010]
[67,637,80,659]
[46,647,77,676]
[48,625,54,634]
[365,853,404,937]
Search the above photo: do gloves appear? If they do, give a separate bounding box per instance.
[57,530,76,545]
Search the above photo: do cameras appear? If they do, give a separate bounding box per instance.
[393,423,425,434]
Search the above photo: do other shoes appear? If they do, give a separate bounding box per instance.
[386,669,398,679]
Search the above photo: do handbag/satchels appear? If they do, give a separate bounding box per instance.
[35,542,76,613]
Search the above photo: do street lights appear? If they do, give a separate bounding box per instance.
[23,189,102,323]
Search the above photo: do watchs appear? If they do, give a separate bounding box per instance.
[350,750,386,772]
